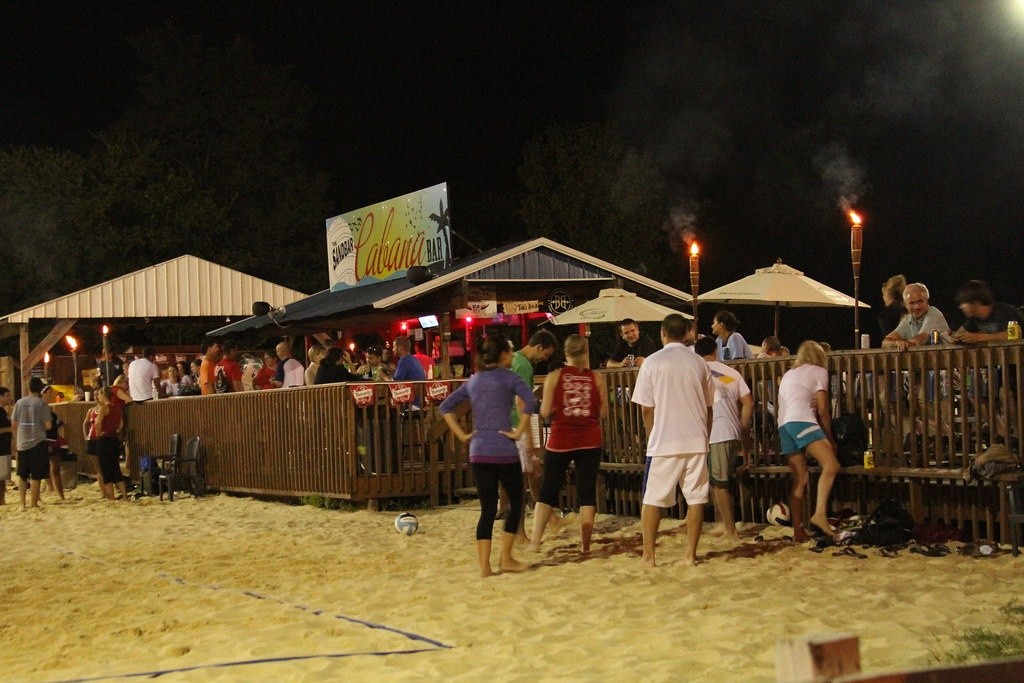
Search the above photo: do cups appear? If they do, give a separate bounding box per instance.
[860,334,870,350]
[863,451,874,469]
[1007,320,1019,340]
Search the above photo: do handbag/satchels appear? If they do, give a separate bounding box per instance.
[177,385,199,395]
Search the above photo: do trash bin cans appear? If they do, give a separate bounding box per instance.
[59,453,77,490]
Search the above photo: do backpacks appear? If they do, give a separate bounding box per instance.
[843,500,916,549]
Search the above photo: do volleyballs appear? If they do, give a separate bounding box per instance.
[394,512,419,536]
[766,502,791,526]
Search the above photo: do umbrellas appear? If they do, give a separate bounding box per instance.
[688,258,871,338]
[537,288,694,325]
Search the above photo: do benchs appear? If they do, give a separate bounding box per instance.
[598,460,1024,551]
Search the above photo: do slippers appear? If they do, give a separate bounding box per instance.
[812,519,1006,559]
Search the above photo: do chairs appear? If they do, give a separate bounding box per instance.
[142,432,203,500]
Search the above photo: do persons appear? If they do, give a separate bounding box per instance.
[630,313,717,566]
[507,330,561,544]
[711,311,837,399]
[879,275,1024,453]
[693,336,755,543]
[606,318,657,367]
[82,336,436,403]
[0,377,65,509]
[777,341,840,544]
[523,334,609,555]
[82,374,133,501]
[438,334,537,577]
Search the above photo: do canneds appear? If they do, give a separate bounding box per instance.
[863,451,874,468]
[1007,321,1019,340]
[627,355,634,367]
[930,328,940,345]
[861,334,870,348]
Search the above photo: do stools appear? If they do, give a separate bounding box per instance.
[400,408,427,464]
